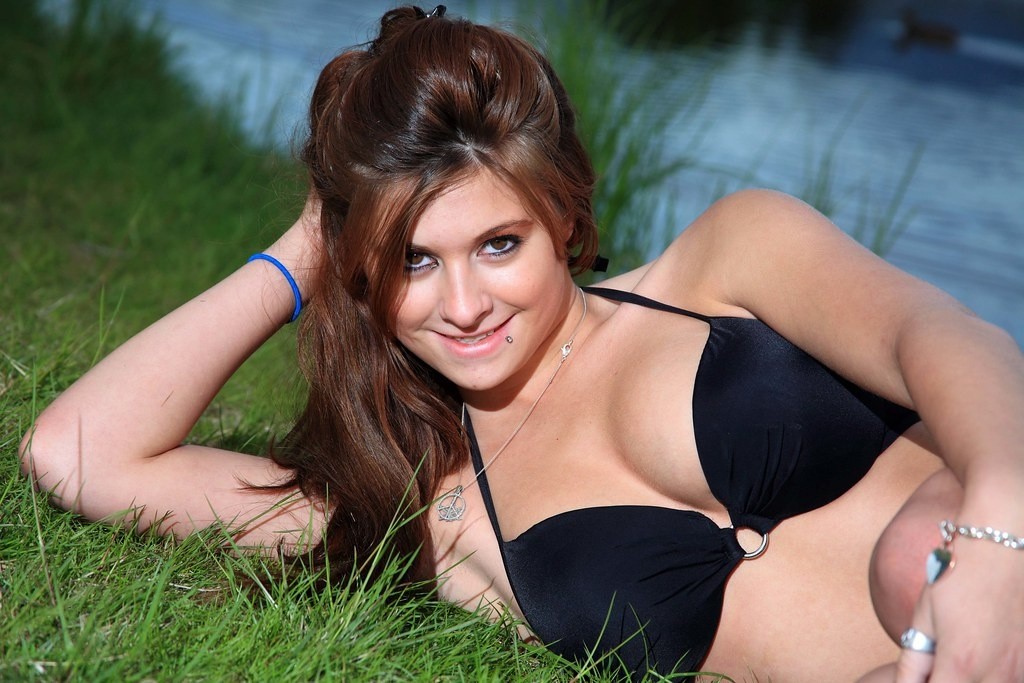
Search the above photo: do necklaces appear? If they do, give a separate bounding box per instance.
[436,287,587,521]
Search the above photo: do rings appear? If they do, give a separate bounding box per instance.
[900,626,936,654]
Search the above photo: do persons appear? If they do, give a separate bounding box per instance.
[20,1,1024,683]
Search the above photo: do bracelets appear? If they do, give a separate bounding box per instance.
[245,252,301,324]
[924,519,1024,584]
